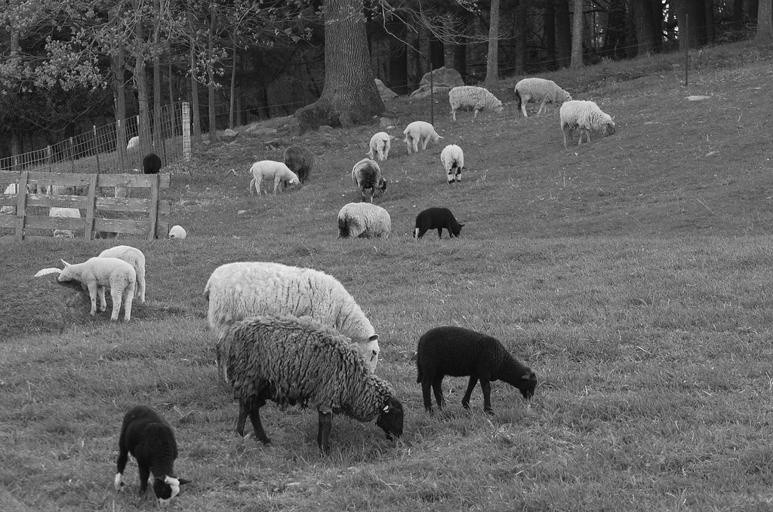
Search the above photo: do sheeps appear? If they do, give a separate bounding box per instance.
[448,85,505,122]
[1,178,136,240]
[514,76,572,118]
[126,136,140,149]
[412,207,465,239]
[336,202,391,241]
[560,100,616,149]
[440,144,467,185]
[249,159,301,198]
[113,405,192,507]
[34,245,147,324]
[142,151,162,175]
[351,157,387,203]
[216,314,405,457]
[283,143,313,188]
[415,325,538,417]
[404,120,443,155]
[168,224,186,242]
[365,131,391,161]
[202,261,379,384]
